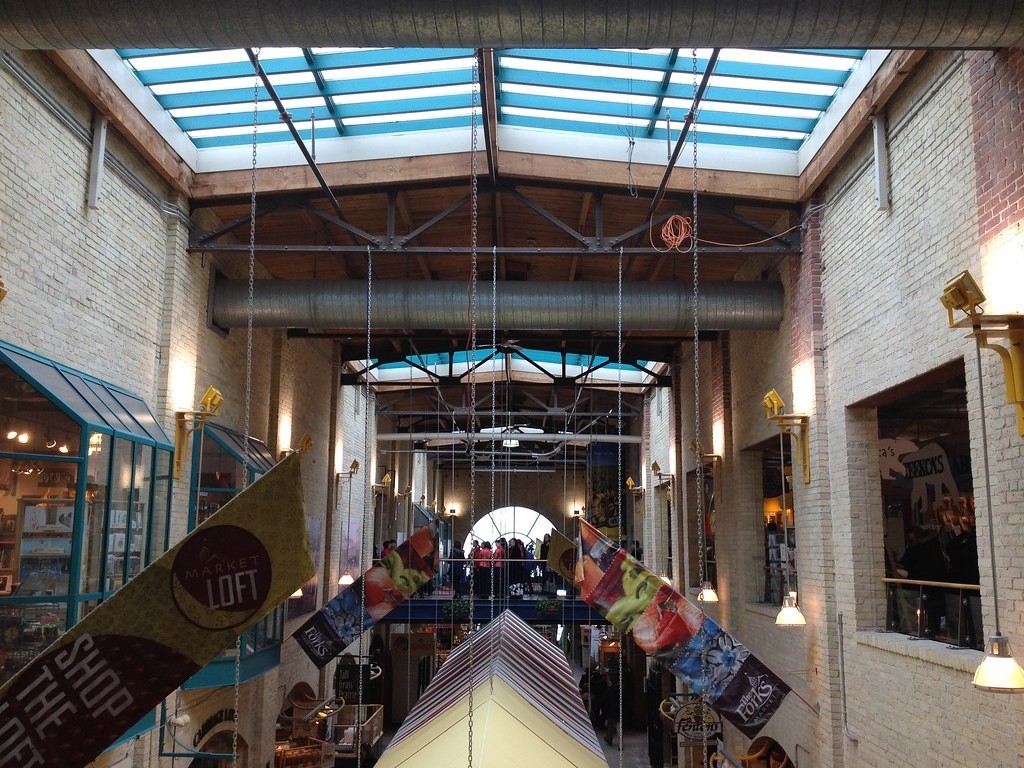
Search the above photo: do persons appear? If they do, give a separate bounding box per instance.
[379,534,563,597]
[579,666,623,751]
[896,515,976,638]
[631,541,645,562]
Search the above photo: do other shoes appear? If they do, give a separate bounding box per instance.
[604,737,613,746]
[453,592,524,599]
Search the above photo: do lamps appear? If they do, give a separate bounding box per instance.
[690,436,722,604]
[650,461,675,587]
[336,460,361,585]
[626,477,646,559]
[940,270,1024,694]
[373,472,391,561]
[280,434,314,598]
[174,387,224,479]
[763,389,811,626]
